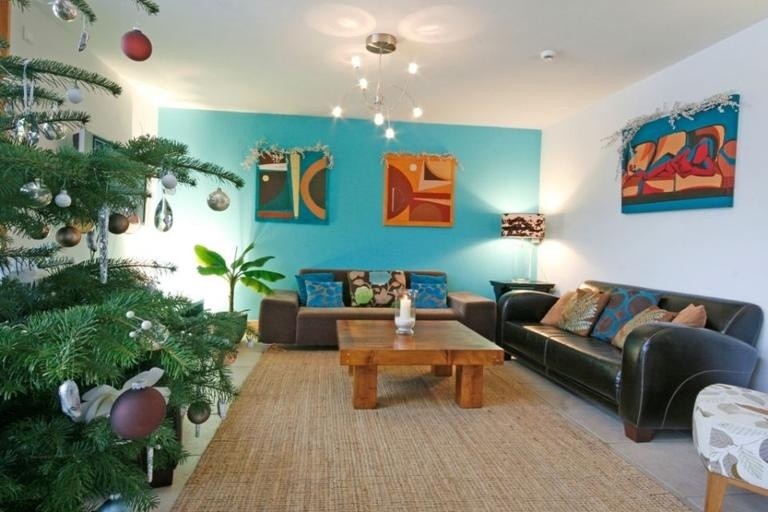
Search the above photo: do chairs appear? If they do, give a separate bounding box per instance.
[691,381,767,511]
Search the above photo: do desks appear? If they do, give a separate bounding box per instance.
[488,278,555,301]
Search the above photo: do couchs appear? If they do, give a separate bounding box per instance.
[496,281,764,442]
[257,269,496,347]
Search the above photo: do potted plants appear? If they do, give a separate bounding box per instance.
[193,241,285,341]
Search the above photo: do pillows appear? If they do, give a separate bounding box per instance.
[591,286,662,341]
[541,290,576,326]
[559,288,610,337]
[295,272,334,306]
[409,273,448,307]
[611,305,676,350]
[671,303,707,328]
[304,279,346,308]
[346,270,407,308]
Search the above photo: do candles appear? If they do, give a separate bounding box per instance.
[399,295,411,318]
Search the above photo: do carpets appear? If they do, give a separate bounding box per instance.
[165,345,699,511]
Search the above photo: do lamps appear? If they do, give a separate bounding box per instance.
[499,212,545,281]
[331,33,424,139]
